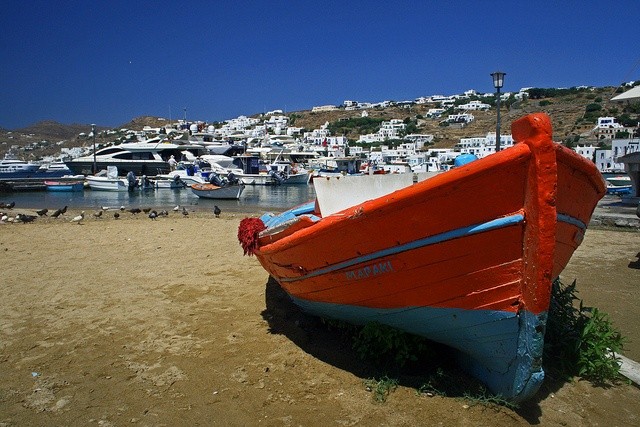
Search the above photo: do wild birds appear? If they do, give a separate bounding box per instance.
[36,209,48,218]
[148,210,168,221]
[50,208,61,219]
[69,211,85,225]
[129,208,141,215]
[114,213,119,220]
[182,207,189,218]
[0,201,15,209]
[61,206,67,215]
[214,205,221,218]
[173,204,180,212]
[93,211,103,219]
[143,208,151,214]
[101,206,110,212]
[120,206,126,213]
[0,212,38,224]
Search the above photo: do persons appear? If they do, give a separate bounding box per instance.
[181,154,186,161]
[126,169,135,179]
[168,155,177,172]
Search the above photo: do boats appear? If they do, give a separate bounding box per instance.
[247,113,608,399]
[85,173,155,191]
[606,176,631,186]
[0,126,389,185]
[44,180,84,191]
[191,171,246,199]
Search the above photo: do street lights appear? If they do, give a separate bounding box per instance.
[490,72,506,151]
